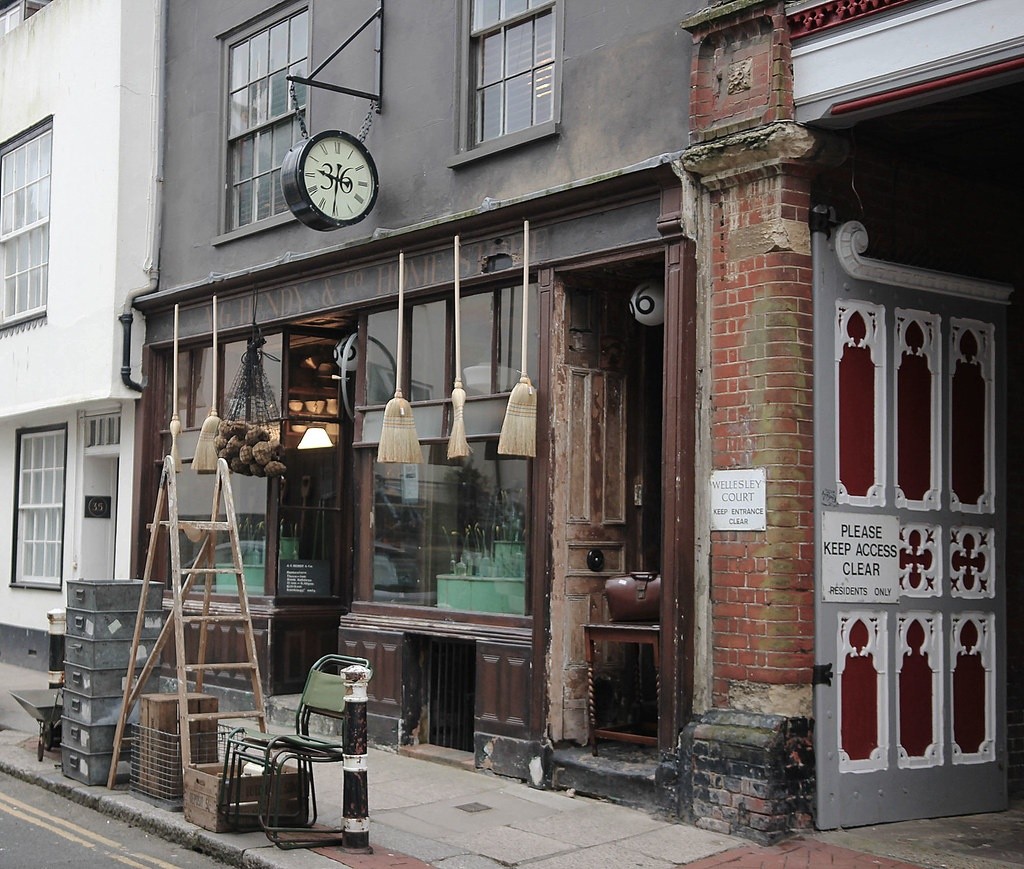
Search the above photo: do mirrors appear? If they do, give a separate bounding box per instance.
[280,128,381,232]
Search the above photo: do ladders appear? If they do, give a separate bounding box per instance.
[106,455,269,790]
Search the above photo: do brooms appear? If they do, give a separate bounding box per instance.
[190,294,233,473]
[496,219,536,459]
[169,303,182,474]
[445,235,476,460]
[375,252,425,464]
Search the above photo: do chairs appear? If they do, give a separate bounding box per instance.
[221,653,374,849]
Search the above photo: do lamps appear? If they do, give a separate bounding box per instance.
[297,427,334,449]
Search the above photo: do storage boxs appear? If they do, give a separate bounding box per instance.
[61,715,132,753]
[63,661,159,699]
[138,692,218,795]
[60,743,132,786]
[182,761,310,832]
[66,579,165,610]
[63,634,162,669]
[65,607,164,639]
[62,687,139,724]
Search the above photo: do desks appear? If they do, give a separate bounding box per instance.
[580,622,662,758]
